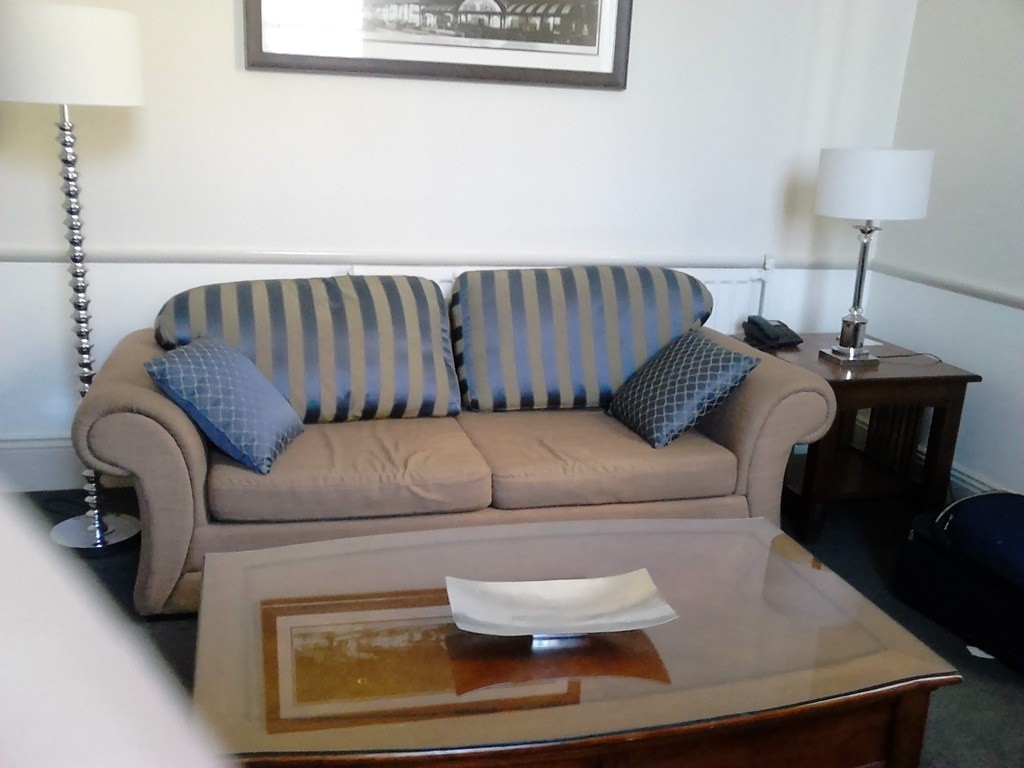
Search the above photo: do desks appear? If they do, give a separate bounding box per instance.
[730,329,982,568]
[195,516,963,768]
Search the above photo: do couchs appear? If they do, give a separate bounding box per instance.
[72,265,838,621]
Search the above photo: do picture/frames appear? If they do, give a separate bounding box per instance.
[244,0,634,88]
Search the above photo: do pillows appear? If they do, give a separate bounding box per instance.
[144,334,306,475]
[608,331,763,450]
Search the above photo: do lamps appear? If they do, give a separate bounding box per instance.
[0,0,149,558]
[815,145,933,369]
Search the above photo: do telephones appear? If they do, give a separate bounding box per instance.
[741,315,804,351]
